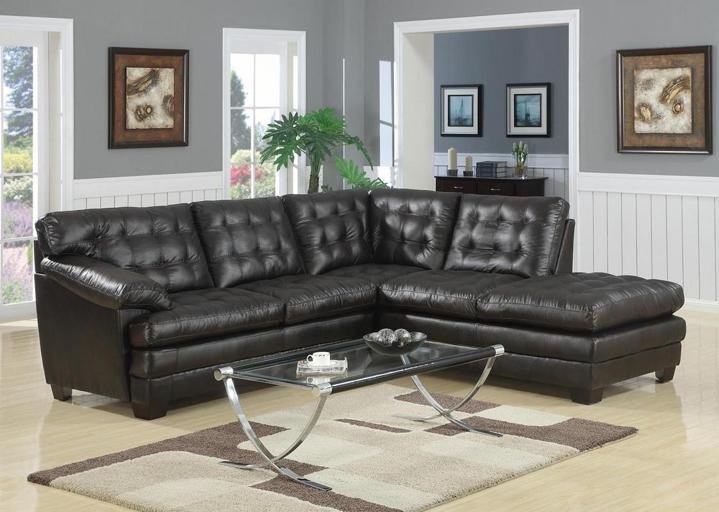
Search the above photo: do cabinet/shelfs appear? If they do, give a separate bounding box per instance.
[434,175,549,196]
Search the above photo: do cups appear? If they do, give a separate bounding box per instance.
[305,378,332,386]
[306,351,330,367]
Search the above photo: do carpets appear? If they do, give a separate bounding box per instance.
[23,382,638,512]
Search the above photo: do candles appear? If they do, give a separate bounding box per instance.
[447,147,473,171]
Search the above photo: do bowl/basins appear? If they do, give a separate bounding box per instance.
[362,331,428,355]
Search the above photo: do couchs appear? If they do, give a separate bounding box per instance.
[29,186,688,421]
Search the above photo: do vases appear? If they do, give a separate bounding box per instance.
[513,166,528,179]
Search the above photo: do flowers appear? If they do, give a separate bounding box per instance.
[512,142,528,166]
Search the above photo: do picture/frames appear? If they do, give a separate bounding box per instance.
[616,45,714,155]
[108,46,190,148]
[438,82,552,139]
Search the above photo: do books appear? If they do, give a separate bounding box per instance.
[475,160,507,178]
